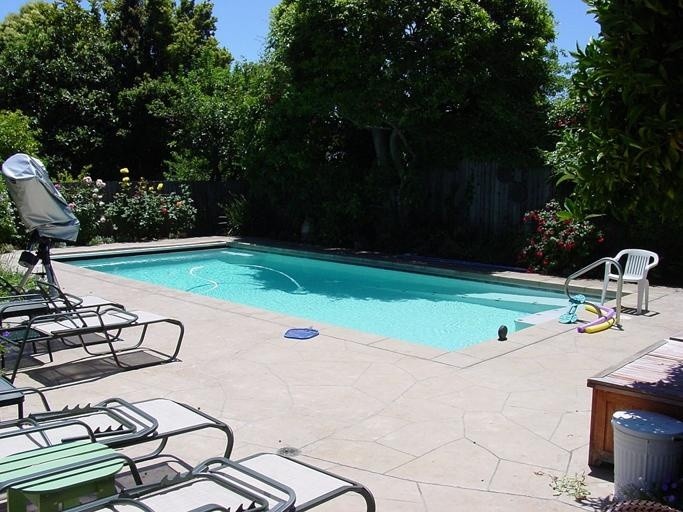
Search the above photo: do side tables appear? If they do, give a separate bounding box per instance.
[0,441,124,512]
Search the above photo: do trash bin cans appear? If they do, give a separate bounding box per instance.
[610,410,683,501]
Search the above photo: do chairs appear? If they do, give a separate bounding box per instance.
[0,398,234,465]
[0,453,376,512]
[601,249,659,315]
[0,281,184,384]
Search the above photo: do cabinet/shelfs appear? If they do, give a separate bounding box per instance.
[587,328,683,466]
